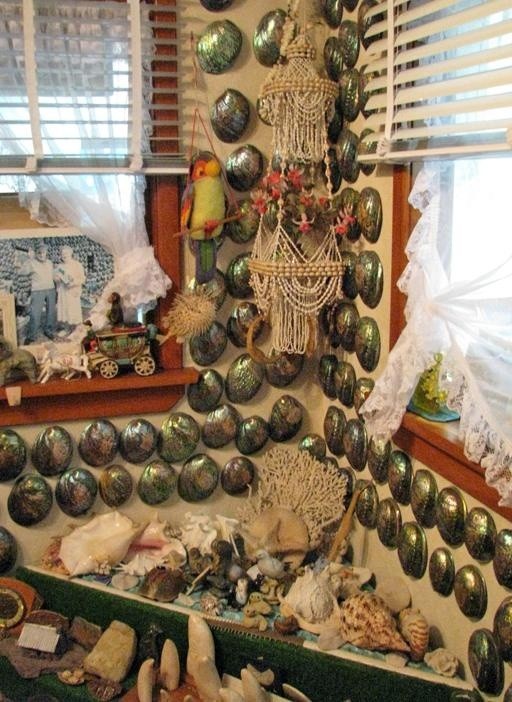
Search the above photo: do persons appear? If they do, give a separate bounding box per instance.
[18,244,55,345]
[54,245,85,335]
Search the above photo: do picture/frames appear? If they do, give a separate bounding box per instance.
[0,226,116,359]
[0,293,17,351]
